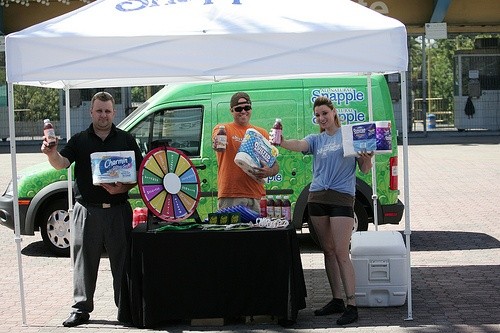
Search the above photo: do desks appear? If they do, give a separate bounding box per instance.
[117,222,307,329]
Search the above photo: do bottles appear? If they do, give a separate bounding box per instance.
[271,117,282,147]
[43,119,57,148]
[259,194,291,224]
[216,125,226,152]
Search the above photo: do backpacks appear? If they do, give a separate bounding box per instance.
[464,97,475,119]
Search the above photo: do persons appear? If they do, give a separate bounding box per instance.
[41,92,144,327]
[212,92,280,216]
[270,99,375,325]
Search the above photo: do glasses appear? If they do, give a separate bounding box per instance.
[232,105,252,112]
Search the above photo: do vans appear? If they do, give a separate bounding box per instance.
[0,74,405,253]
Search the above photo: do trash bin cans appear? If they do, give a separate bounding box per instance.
[427,114,436,129]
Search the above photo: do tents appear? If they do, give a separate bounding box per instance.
[4,0,413,328]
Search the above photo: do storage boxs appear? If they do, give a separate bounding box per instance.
[351,231,407,307]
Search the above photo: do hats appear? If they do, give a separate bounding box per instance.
[230,92,251,108]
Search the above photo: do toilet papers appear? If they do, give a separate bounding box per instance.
[341,119,391,159]
[87,149,141,184]
[236,129,277,180]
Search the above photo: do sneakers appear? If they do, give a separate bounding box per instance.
[336,305,358,325]
[314,298,344,316]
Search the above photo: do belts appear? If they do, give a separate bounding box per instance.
[84,202,123,209]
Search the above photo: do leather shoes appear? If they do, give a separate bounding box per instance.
[62,313,90,327]
